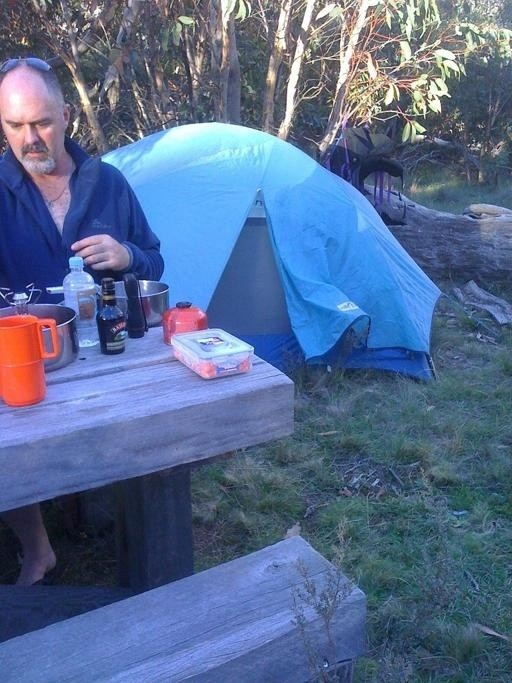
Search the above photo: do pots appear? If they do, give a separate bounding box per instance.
[46,280,170,329]
[0,302,80,373]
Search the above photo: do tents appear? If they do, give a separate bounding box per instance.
[102,122,446,385]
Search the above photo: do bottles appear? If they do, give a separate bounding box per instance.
[163,301,208,346]
[63,257,101,348]
[96,278,128,356]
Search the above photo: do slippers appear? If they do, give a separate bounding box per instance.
[4,568,57,624]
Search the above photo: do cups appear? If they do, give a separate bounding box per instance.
[0,315,61,407]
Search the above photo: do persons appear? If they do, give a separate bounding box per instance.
[0,58,164,586]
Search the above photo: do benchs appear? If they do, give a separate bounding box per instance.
[0,535,366,682]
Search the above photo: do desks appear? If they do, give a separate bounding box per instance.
[1,302,294,585]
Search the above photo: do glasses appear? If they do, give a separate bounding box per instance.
[1,56,52,77]
